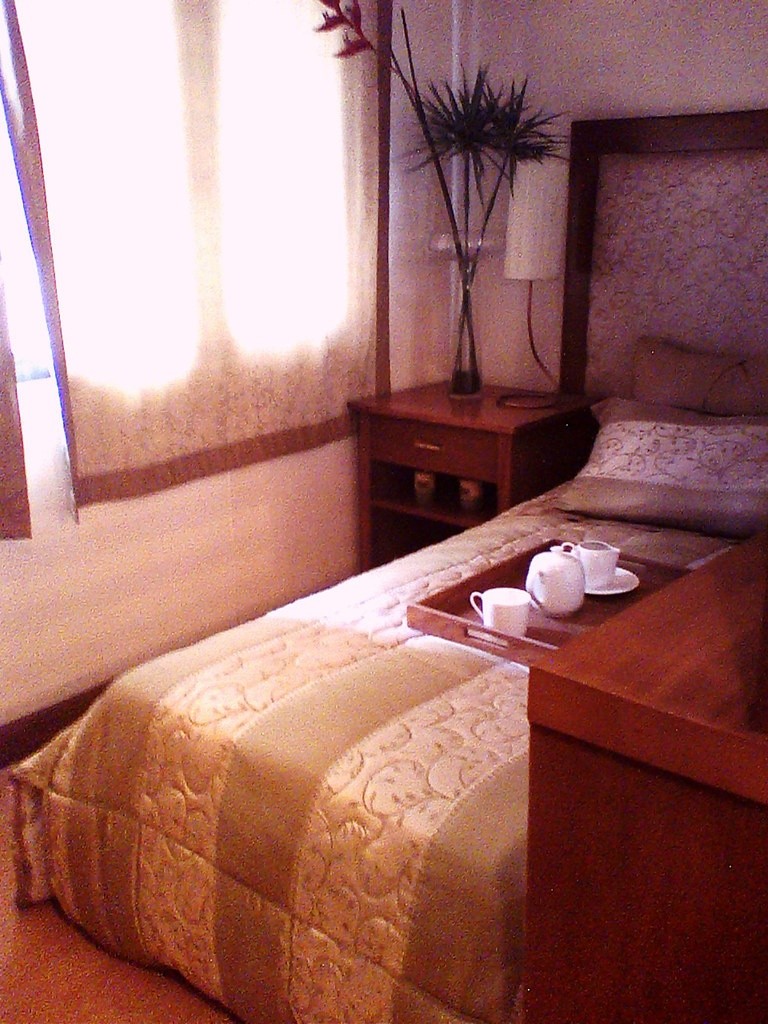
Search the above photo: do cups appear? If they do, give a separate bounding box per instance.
[562,541,620,587]
[470,588,531,648]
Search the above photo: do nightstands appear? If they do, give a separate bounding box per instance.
[344,380,603,575]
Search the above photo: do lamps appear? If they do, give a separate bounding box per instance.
[507,135,566,387]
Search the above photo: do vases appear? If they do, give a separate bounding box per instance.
[431,230,507,404]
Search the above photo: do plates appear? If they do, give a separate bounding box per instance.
[586,568,639,594]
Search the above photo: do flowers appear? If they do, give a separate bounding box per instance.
[313,4,575,392]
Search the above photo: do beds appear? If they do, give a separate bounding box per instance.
[8,442,767,1022]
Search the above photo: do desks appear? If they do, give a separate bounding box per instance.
[406,528,768,1023]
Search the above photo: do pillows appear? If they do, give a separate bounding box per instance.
[551,400,768,539]
[630,332,767,433]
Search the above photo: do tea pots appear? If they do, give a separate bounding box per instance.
[526,545,586,619]
